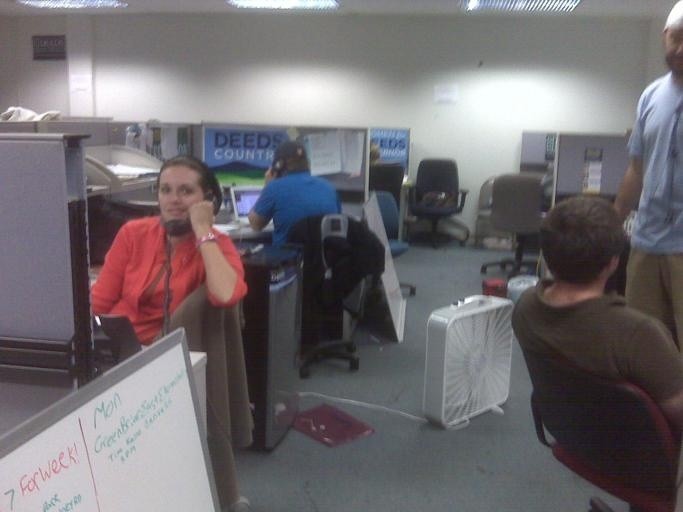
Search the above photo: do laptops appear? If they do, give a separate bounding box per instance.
[229,185,273,227]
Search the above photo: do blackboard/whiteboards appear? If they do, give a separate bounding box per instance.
[0,327,221,512]
[364,191,407,343]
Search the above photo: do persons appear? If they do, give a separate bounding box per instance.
[238,139,342,276]
[605,1,683,356]
[511,194,681,454]
[86,152,250,350]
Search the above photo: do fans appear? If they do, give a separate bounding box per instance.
[422,293,516,433]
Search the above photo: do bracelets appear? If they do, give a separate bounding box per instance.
[193,232,217,249]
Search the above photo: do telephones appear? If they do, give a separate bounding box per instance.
[271,157,284,177]
[165,194,219,235]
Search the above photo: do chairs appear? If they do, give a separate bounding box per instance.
[519,347,680,512]
[476,173,552,279]
[408,159,468,250]
[291,209,382,378]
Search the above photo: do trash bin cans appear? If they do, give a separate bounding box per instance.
[508,275,538,303]
